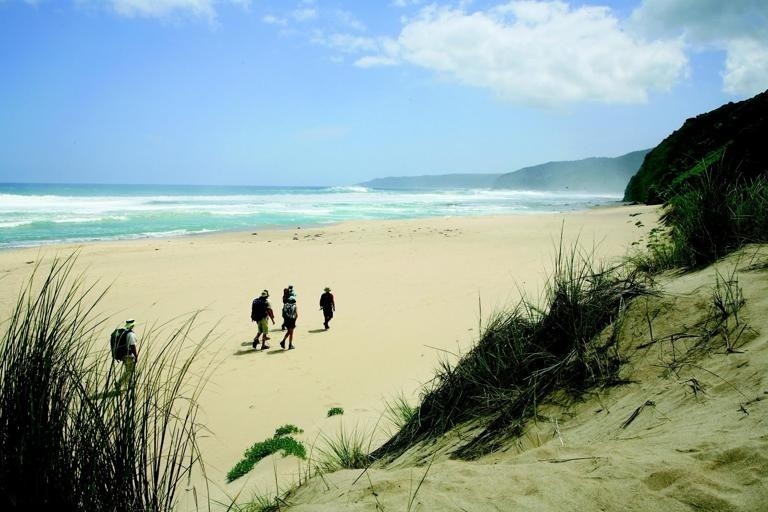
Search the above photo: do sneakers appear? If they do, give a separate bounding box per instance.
[280,342,295,350]
[253,336,271,350]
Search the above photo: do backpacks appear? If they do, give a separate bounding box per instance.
[251,298,267,321]
[110,328,134,361]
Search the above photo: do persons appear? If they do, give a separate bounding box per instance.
[258,289,272,341]
[281,285,295,331]
[251,291,275,351]
[107,318,140,394]
[319,288,335,330]
[288,286,296,302]
[279,297,298,349]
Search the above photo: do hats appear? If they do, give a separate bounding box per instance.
[123,319,136,329]
[261,290,269,297]
[323,287,331,291]
[288,296,296,300]
